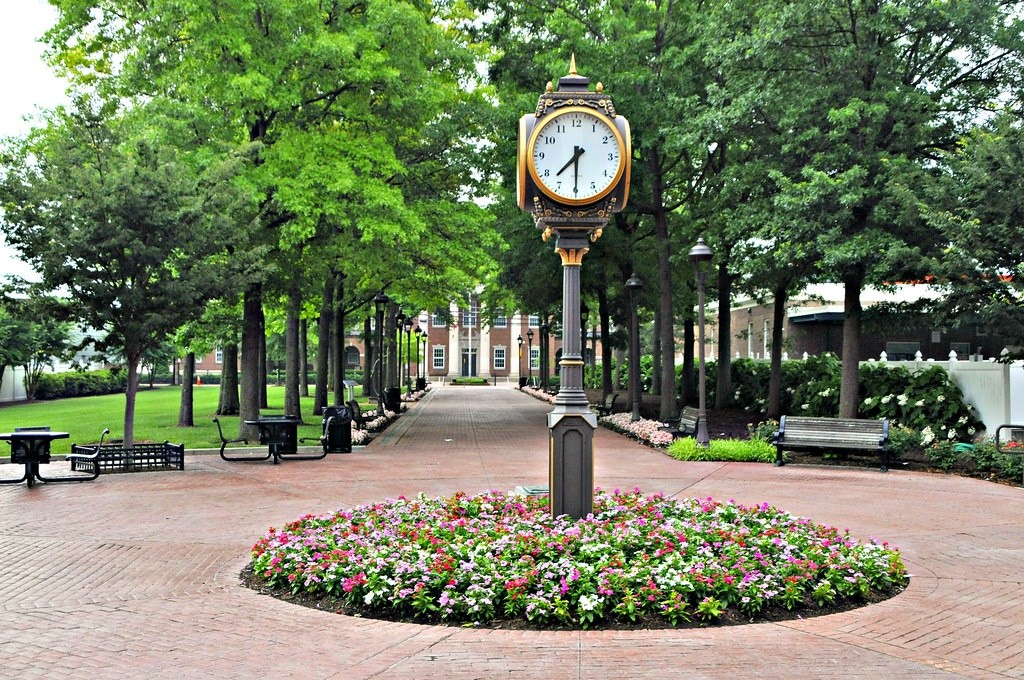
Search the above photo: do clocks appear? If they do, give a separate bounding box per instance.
[527,105,627,207]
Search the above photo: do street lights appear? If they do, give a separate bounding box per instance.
[517,335,524,383]
[414,325,422,391]
[526,328,534,387]
[624,270,644,422]
[404,317,414,399]
[579,301,591,390]
[395,309,406,389]
[421,331,428,390]
[687,234,716,449]
[539,320,552,394]
[373,290,386,417]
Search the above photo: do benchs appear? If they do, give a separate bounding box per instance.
[532,380,560,396]
[345,399,378,431]
[589,393,620,421]
[772,415,890,473]
[409,384,420,395]
[658,405,711,439]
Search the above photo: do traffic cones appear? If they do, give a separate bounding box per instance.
[196,375,202,385]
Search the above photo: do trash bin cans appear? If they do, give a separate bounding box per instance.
[321,405,352,454]
[519,377,527,390]
[416,378,426,391]
[268,415,297,456]
[384,387,400,414]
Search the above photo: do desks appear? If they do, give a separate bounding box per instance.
[244,417,300,464]
[0,431,70,488]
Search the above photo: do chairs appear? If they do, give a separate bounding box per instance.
[36,428,110,483]
[213,415,336,461]
